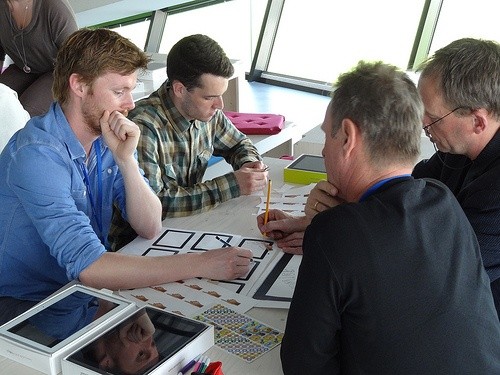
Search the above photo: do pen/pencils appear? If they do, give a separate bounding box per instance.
[264,179,272,239]
[177,354,210,375]
[216,236,255,262]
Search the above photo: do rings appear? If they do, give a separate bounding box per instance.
[314,201,320,210]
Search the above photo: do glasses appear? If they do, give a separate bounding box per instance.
[423,106,473,135]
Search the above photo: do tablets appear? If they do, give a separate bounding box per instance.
[0,286,129,354]
[65,305,210,375]
[288,155,327,172]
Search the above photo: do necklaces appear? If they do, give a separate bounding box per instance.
[9,0,31,73]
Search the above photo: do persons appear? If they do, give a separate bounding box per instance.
[0,0,79,118]
[107,34,269,253]
[29,290,160,375]
[257,36,500,323]
[280,61,499,375]
[0,25,253,326]
